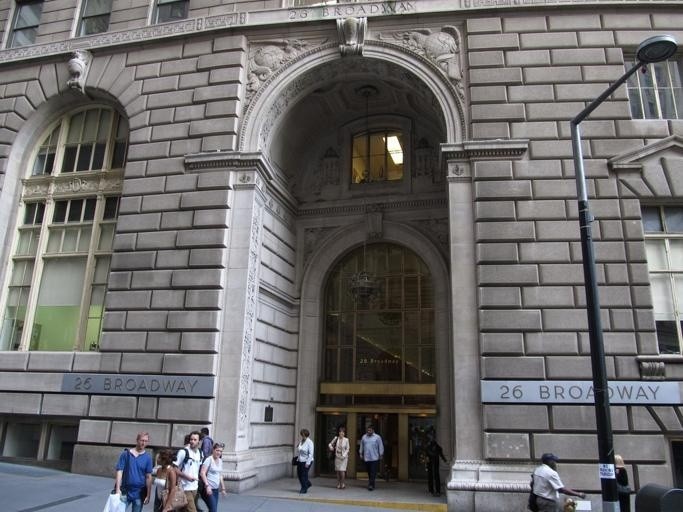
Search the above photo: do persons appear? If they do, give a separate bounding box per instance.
[425,440,449,497]
[327,427,350,489]
[359,425,385,490]
[295,428,314,494]
[116,427,227,512]
[532,452,586,512]
[615,454,631,512]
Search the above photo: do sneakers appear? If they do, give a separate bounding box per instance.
[300,481,376,494]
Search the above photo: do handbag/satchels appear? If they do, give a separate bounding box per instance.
[327,447,335,462]
[198,465,208,495]
[292,456,300,465]
[103,494,126,512]
[111,484,128,496]
[159,485,190,512]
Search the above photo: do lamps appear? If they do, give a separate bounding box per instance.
[383,136,403,165]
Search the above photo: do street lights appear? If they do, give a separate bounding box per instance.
[568,35,680,512]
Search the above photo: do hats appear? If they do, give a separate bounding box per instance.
[541,452,558,462]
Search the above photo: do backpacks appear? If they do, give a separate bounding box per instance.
[165,447,189,467]
